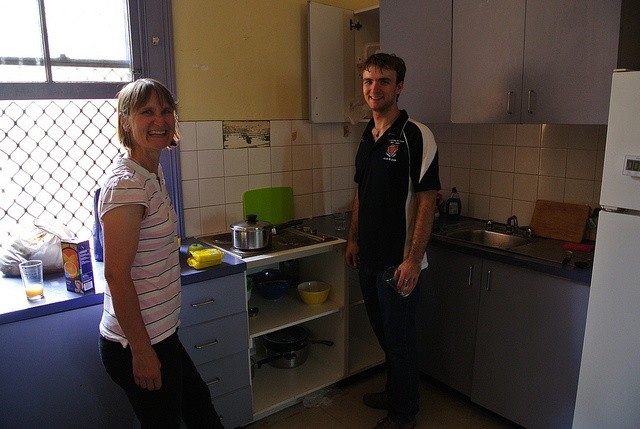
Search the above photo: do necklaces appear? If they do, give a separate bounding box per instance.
[128,155,160,187]
[373,112,403,138]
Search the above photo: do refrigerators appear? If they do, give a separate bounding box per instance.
[572,68,640,429]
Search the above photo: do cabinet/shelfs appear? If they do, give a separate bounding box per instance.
[348,267,386,377]
[244,242,348,421]
[415,239,592,429]
[177,271,254,429]
[450,0,640,125]
[307,0,453,125]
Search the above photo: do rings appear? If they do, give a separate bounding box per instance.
[404,279,409,282]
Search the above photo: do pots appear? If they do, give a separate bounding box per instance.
[259,324,334,352]
[229,215,274,250]
[257,344,309,369]
[252,269,293,300]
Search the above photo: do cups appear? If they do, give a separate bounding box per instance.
[382,266,414,298]
[333,213,350,230]
[19,260,44,303]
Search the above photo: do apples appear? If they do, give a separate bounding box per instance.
[187,243,204,256]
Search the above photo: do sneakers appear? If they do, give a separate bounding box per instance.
[373,417,417,428]
[363,393,390,409]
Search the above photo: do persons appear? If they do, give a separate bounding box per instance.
[344,51,442,428]
[95,77,226,427]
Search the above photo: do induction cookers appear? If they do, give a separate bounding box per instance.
[197,222,339,259]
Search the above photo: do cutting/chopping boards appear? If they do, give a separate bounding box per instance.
[529,199,591,243]
[242,186,294,225]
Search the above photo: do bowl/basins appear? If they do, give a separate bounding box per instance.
[298,281,330,305]
[246,277,254,301]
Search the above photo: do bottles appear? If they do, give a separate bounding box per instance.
[446,187,462,220]
[433,205,439,220]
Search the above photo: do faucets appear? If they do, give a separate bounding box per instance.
[505,215,518,233]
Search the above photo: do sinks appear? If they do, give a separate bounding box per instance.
[441,226,534,250]
